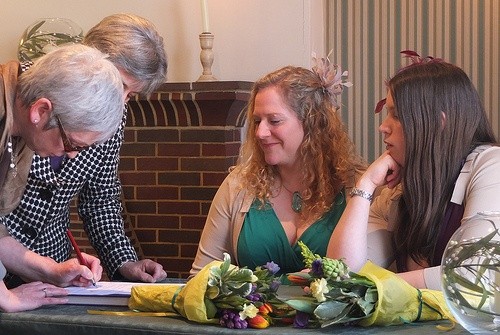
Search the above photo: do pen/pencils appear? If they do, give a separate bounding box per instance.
[66,228,96,287]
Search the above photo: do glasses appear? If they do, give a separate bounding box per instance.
[54,114,97,153]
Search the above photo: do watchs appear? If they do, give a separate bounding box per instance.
[349,186,374,202]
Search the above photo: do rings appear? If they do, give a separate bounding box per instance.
[43,289,48,297]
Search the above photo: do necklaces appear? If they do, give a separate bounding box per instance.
[279,179,305,213]
[4,135,21,181]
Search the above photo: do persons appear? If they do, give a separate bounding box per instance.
[325,60,500,319]
[0,43,128,314]
[0,13,168,289]
[189,65,368,287]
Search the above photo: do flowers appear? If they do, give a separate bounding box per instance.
[375,50,444,113]
[311,48,354,110]
[86,240,491,331]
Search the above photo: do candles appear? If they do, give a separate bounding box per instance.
[200,0,211,32]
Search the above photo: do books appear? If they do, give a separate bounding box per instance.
[51,282,131,307]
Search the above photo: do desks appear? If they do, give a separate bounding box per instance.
[0,304,472,335]
